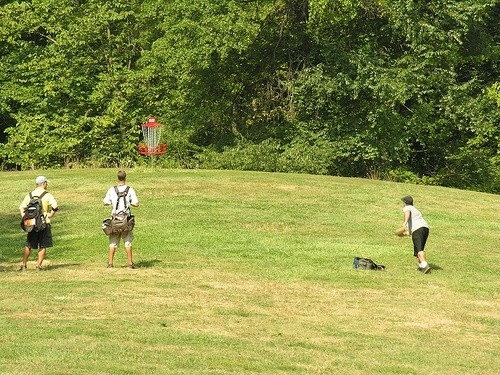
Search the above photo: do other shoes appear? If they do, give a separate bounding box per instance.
[107,264,113,268]
[35,266,43,271]
[129,264,136,269]
[20,266,27,271]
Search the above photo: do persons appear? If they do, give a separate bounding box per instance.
[103,170,139,268]
[16,176,58,272]
[400,195,431,274]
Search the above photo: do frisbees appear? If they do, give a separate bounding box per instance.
[393,228,406,235]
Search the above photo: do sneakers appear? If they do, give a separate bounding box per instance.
[417,264,430,274]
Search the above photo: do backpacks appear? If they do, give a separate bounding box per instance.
[102,186,135,235]
[21,191,48,232]
[354,258,385,270]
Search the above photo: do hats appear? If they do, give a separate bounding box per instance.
[401,196,413,205]
[36,176,48,184]
[117,171,126,177]
